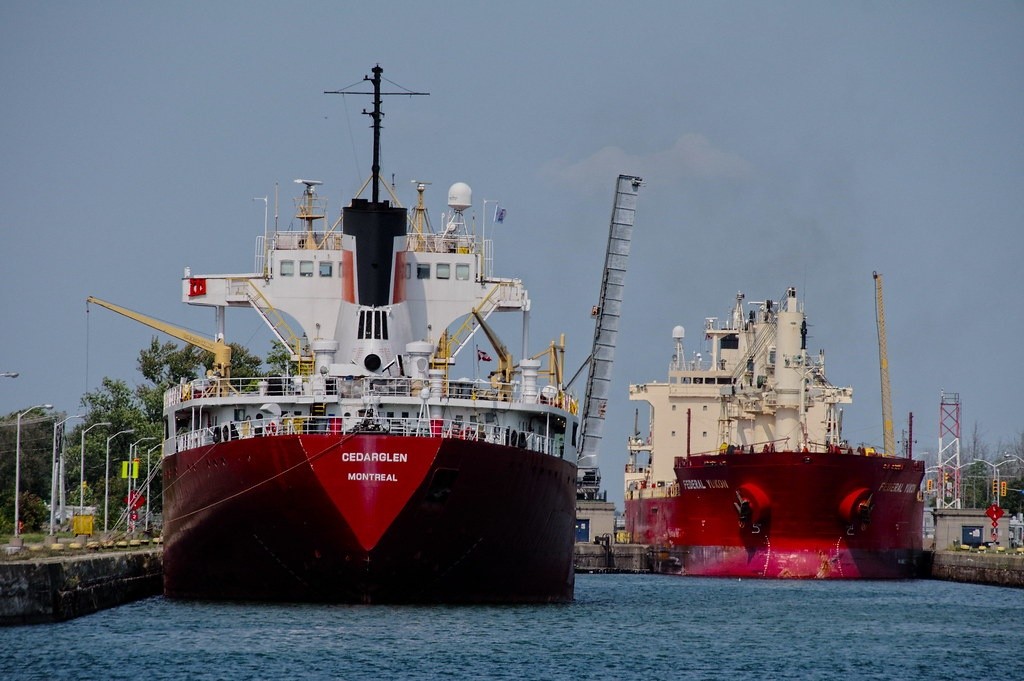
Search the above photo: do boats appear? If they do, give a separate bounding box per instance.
[625,267,922,579]
[146,63,579,605]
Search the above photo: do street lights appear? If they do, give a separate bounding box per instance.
[126,437,157,532]
[105,429,136,533]
[911,451,978,510]
[14,403,53,538]
[1005,452,1024,465]
[145,443,162,532]
[81,422,111,515]
[50,413,86,533]
[975,458,1017,507]
[0,371,19,379]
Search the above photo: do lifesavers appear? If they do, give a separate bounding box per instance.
[267,422,276,436]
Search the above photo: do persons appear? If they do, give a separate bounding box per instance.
[795,446,808,452]
[494,425,500,439]
[763,443,775,453]
[860,444,866,455]
[230,421,235,438]
[506,426,510,445]
[18,521,24,534]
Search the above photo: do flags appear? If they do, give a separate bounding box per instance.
[495,207,506,224]
[478,350,492,360]
